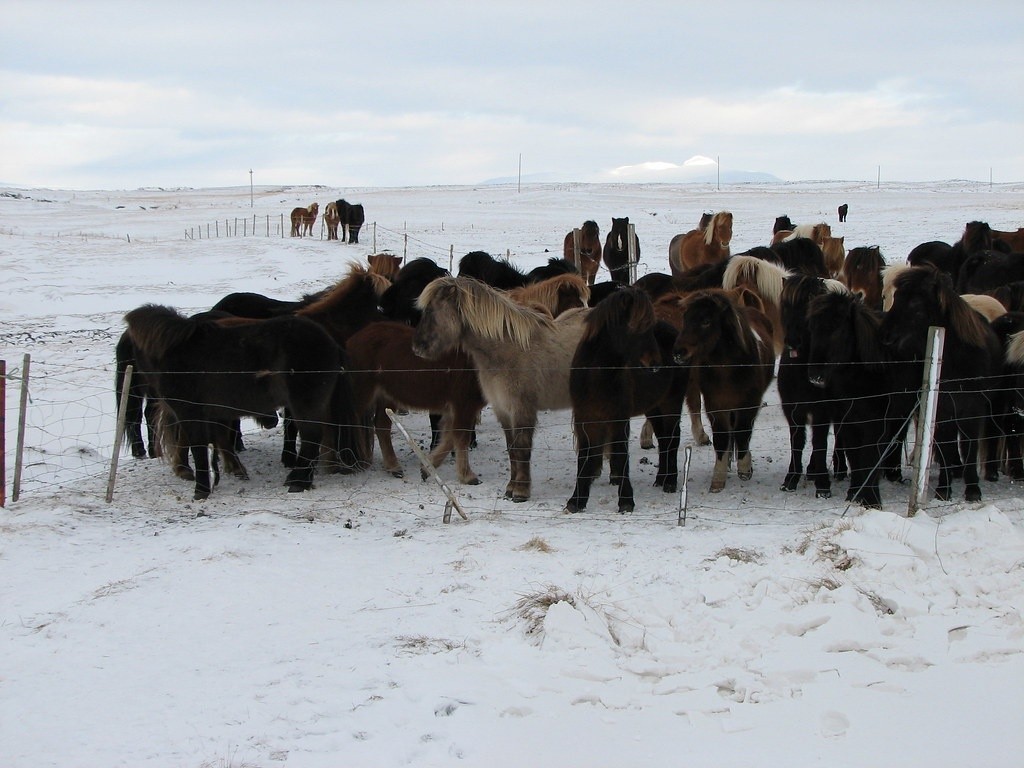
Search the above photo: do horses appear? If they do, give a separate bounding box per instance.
[772,215,797,234]
[668,213,733,277]
[336,198,364,246]
[113,221,1024,512]
[699,213,716,231]
[324,202,340,240]
[290,203,319,236]
[603,217,641,285]
[563,219,602,285]
[837,204,848,222]
